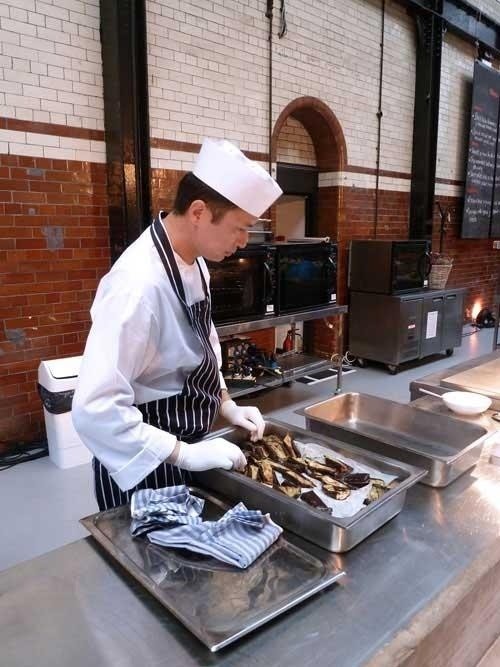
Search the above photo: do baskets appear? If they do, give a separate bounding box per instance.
[427,264,453,290]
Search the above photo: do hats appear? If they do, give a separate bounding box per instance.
[193,136,284,218]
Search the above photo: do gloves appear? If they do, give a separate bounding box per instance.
[219,399,266,443]
[176,437,247,472]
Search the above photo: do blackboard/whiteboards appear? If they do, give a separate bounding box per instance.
[461,59,500,239]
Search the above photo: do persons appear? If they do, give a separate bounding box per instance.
[72,139,284,513]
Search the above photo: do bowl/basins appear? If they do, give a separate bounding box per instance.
[442,392,492,416]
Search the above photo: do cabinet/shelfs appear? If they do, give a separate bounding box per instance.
[215,304,348,397]
[349,288,465,374]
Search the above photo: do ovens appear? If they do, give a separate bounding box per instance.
[205,244,266,325]
[277,241,337,313]
[395,287,464,364]
[352,240,431,294]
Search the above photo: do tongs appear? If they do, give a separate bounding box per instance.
[288,236,330,243]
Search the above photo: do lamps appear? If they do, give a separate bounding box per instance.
[475,307,495,329]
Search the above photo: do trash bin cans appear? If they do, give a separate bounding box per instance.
[38,356,92,469]
